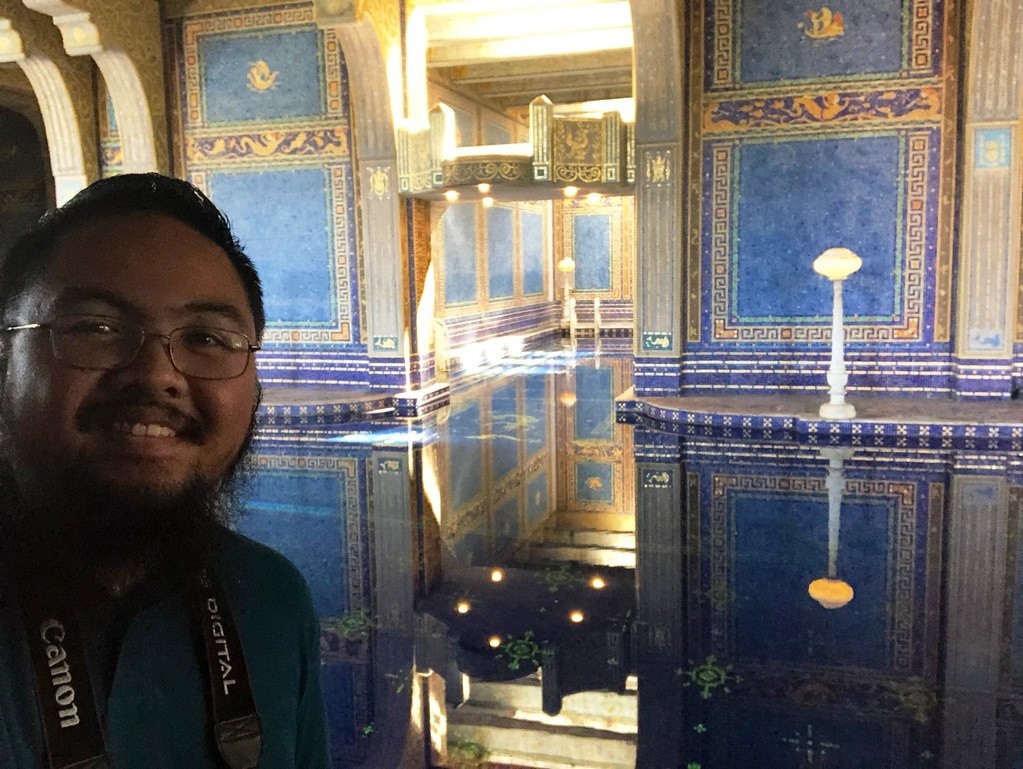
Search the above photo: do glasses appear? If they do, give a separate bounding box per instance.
[5,313,261,380]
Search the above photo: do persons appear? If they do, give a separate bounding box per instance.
[0,173,333,769]
